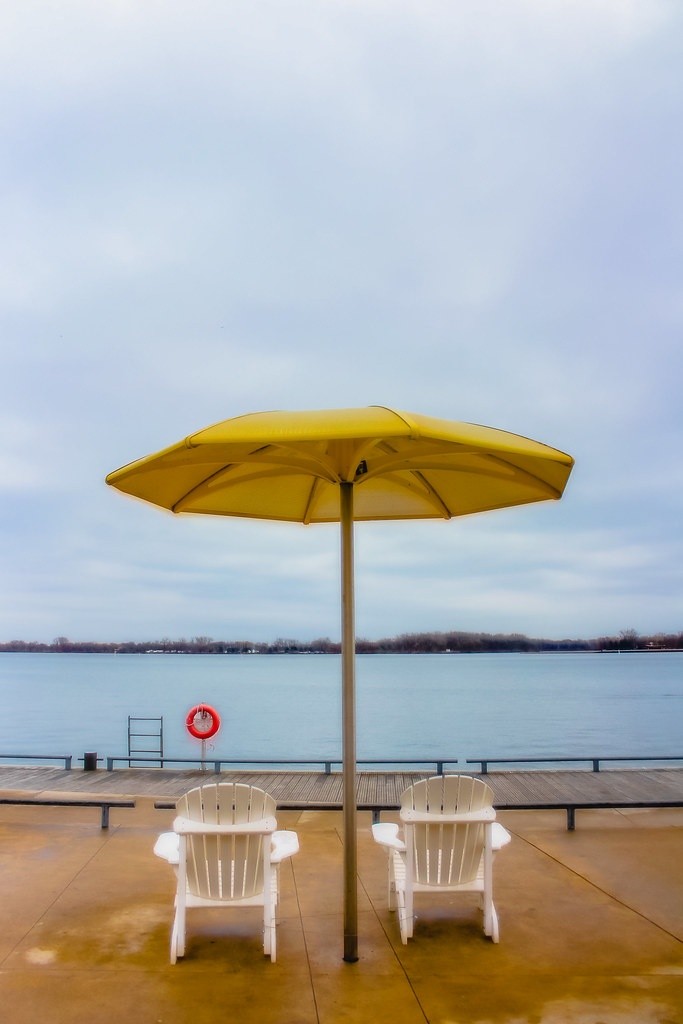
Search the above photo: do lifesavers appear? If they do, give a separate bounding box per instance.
[186,704,221,740]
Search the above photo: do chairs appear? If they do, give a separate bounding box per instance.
[152,783,300,964]
[371,774,511,946]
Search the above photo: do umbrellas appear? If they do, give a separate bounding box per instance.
[106,406,573,963]
